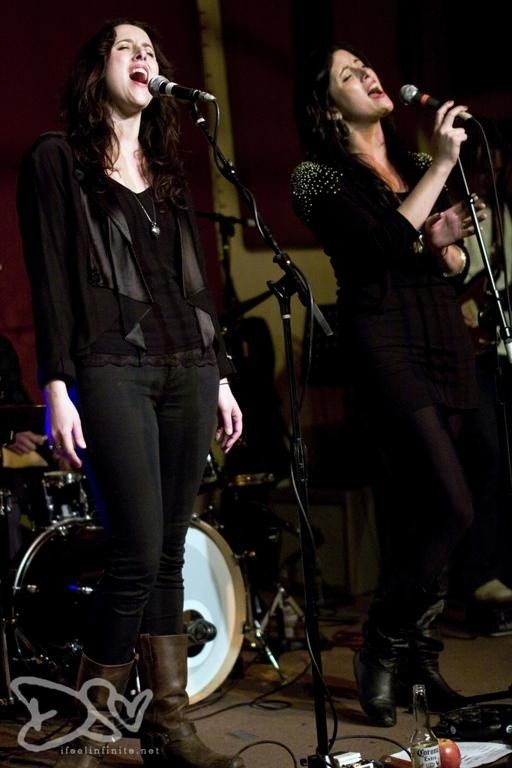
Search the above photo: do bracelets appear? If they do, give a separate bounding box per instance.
[440,247,450,261]
[219,382,229,386]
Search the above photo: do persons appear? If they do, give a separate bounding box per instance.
[13,16,244,767]
[0,334,61,473]
[285,38,480,733]
[455,128,511,605]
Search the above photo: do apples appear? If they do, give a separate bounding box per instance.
[439,739,462,768]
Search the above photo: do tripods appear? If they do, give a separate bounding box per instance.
[251,570,335,649]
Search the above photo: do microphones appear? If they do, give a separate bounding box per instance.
[148,73,217,105]
[398,84,475,123]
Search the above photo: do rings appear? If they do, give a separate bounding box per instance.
[48,439,62,451]
[462,222,470,229]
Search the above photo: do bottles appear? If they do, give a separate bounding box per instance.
[406,685,442,768]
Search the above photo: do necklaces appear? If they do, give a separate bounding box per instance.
[393,193,427,256]
[132,188,161,240]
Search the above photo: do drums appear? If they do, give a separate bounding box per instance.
[218,387,285,488]
[222,317,279,414]
[12,507,248,709]
[301,422,378,500]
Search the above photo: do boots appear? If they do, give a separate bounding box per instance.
[138,631,245,768]
[51,648,140,768]
[352,579,471,727]
